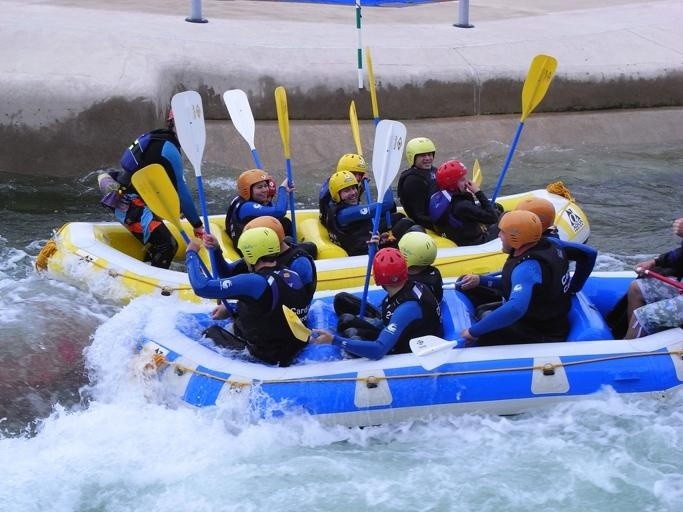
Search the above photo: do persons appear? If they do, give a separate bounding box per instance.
[98,109,205,269]
[623,218,683,339]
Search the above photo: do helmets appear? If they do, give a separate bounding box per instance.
[405,136,436,168]
[435,160,467,192]
[237,168,286,265]
[372,247,408,286]
[329,171,359,203]
[336,153,366,175]
[167,108,174,121]
[516,199,556,229]
[500,210,543,247]
[395,232,438,267]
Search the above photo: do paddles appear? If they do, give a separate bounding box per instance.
[349,48,407,320]
[281,304,343,343]
[409,334,468,371]
[223,86,297,249]
[131,90,237,321]
[489,54,560,208]
[472,158,483,188]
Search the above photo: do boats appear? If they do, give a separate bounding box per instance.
[30,176,594,312]
[106,268,682,425]
